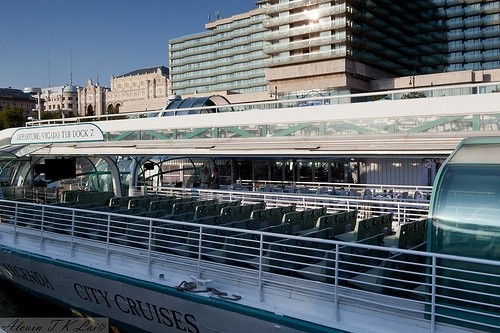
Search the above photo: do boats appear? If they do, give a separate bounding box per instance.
[0,67,500,333]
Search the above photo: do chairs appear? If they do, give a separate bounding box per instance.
[1,184,499,317]
